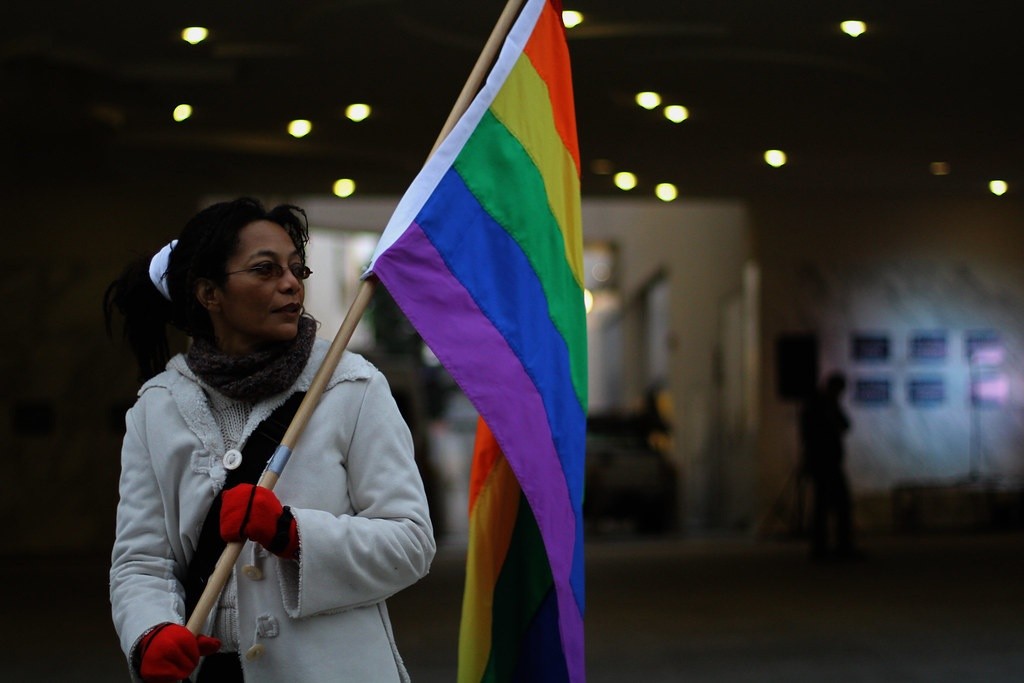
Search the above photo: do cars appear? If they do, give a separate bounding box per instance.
[522,420,681,537]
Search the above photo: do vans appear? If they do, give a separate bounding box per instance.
[361,352,434,524]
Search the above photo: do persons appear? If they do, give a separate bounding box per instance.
[794,370,869,562]
[106,196,437,682]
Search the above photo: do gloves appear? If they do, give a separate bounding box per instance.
[220,482,300,560]
[139,623,222,683]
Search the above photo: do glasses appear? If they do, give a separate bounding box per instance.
[214,262,313,278]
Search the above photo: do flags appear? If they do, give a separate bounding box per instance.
[363,0,589,683]
